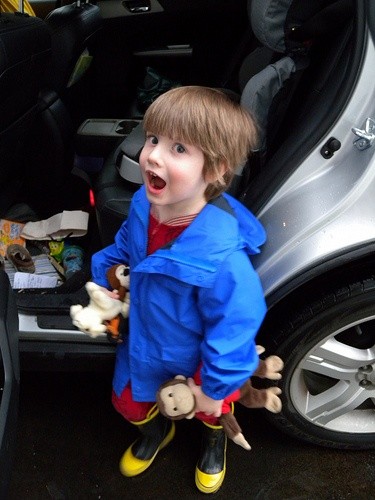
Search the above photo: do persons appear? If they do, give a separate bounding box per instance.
[91,86,268,494]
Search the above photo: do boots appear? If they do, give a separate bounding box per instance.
[194,401,235,493]
[119,405,176,477]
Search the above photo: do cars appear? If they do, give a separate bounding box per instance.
[0,0,375,499]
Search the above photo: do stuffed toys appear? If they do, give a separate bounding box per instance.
[70,281,130,338]
[155,344,284,450]
[106,263,130,343]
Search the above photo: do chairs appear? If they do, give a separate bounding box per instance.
[0,0,299,231]
